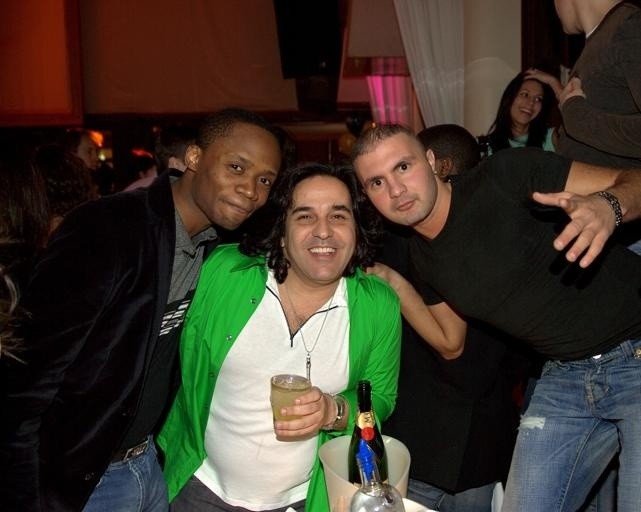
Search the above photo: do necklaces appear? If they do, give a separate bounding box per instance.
[511,123,530,135]
[284,277,341,382]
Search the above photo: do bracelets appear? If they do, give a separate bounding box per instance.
[320,391,346,433]
[586,185,625,227]
[554,86,566,100]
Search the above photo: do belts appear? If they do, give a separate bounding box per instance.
[111,437,151,464]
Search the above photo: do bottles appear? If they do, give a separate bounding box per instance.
[347,380,390,489]
[346,439,405,512]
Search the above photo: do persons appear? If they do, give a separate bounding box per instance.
[550,0,641,170]
[1,123,203,308]
[153,160,406,511]
[2,106,286,512]
[390,124,527,493]
[476,63,569,156]
[346,123,641,510]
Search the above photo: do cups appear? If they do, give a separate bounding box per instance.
[269,373,309,444]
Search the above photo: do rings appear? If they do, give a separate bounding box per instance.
[531,66,536,71]
